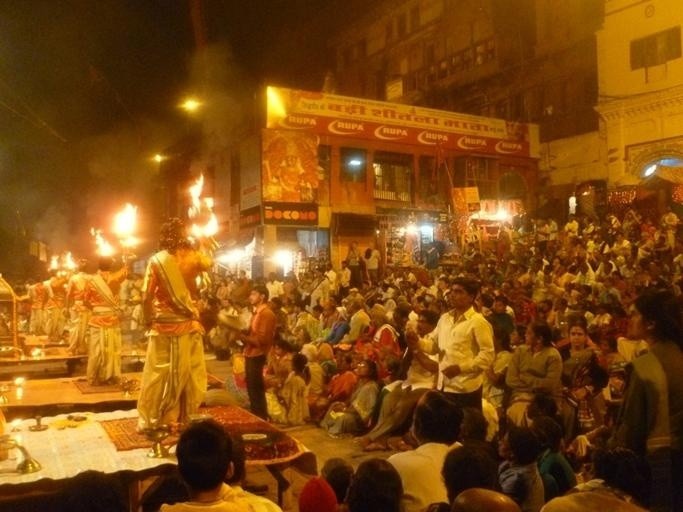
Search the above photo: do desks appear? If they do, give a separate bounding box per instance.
[1,318,316,512]
